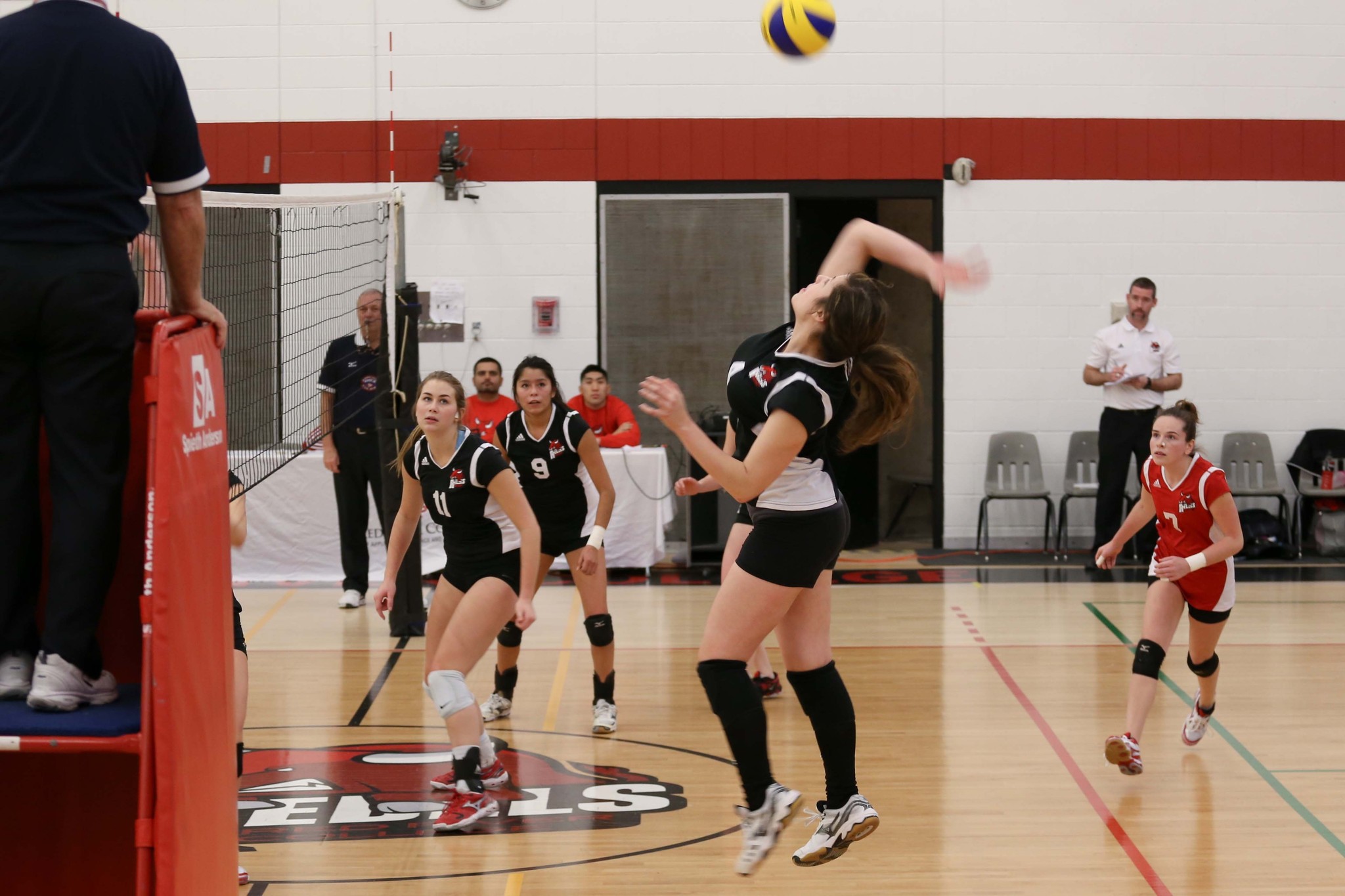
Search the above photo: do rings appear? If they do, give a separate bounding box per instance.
[1116,373,1120,377]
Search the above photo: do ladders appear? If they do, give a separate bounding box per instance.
[0,309,240,896]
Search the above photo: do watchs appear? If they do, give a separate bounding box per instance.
[1143,377,1152,389]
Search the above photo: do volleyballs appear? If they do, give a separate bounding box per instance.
[761,0,835,59]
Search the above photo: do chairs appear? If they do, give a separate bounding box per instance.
[880,465,940,547]
[1066,425,1122,562]
[1295,409,1345,562]
[1213,426,1294,557]
[972,422,1058,570]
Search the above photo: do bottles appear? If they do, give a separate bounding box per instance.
[1321,455,1335,491]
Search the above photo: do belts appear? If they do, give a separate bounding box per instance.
[1105,407,1159,416]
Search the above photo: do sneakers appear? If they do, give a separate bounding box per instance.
[1104,732,1144,776]
[25,649,119,711]
[734,782,803,877]
[430,759,508,790]
[480,693,512,723]
[339,589,365,608]
[0,654,33,698]
[432,786,500,832]
[591,699,617,733]
[793,795,880,868]
[1183,690,1215,745]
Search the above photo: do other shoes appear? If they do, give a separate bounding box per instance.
[239,867,249,886]
[751,670,783,697]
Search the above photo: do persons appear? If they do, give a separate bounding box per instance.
[0,0,230,706]
[212,217,1244,877]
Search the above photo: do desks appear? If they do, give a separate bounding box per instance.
[225,445,681,589]
